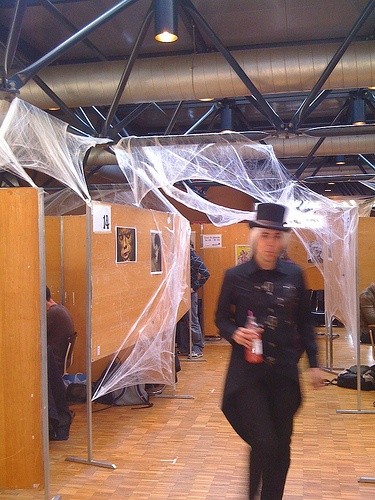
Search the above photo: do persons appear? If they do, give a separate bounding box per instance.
[174,239,211,358]
[45,286,76,442]
[214,203,324,500]
[359,281,375,345]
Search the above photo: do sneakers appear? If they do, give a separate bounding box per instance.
[188,353,204,359]
[176,350,189,356]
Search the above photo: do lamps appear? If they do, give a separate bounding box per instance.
[336,156,345,165]
[352,99,366,125]
[153,0,179,43]
[220,108,234,133]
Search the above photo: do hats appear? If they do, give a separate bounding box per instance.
[248,203,289,232]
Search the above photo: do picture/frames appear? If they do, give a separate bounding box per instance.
[234,245,252,267]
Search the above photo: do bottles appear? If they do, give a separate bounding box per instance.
[245,311,264,364]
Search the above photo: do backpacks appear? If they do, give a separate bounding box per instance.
[322,365,375,391]
[93,357,155,410]
